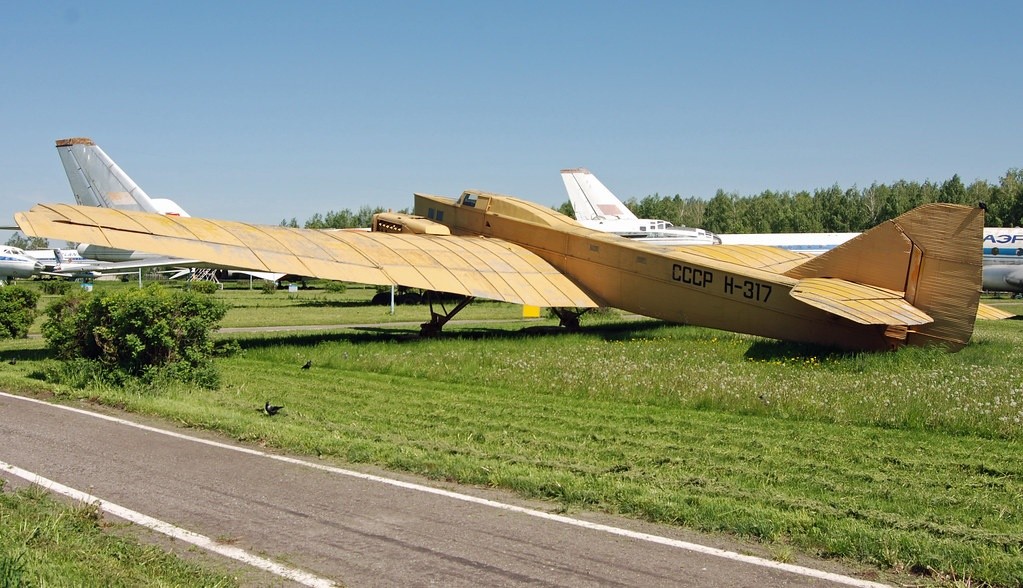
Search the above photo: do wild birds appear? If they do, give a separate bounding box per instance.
[265,402,285,414]
[302,360,312,369]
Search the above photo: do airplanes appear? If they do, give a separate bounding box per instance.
[56,138,722,309]
[556,167,1023,298]
[21,246,107,287]
[0,244,45,284]
[12,186,1023,358]
